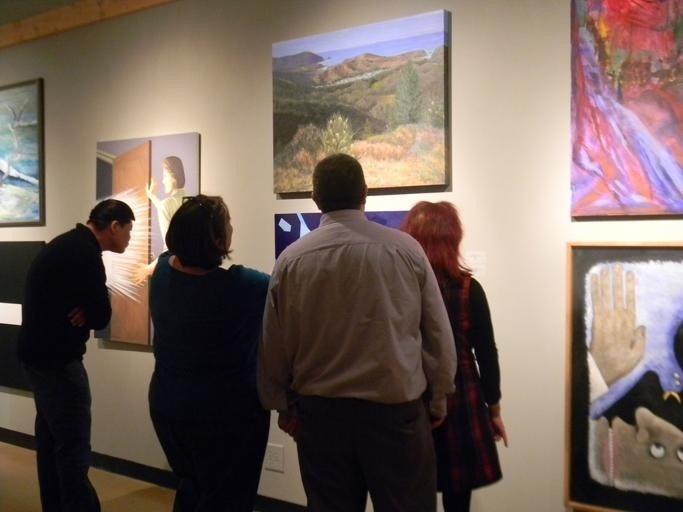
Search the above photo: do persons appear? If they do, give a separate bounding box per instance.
[585,263,683,436]
[149,194,278,512]
[257,151,463,509]
[141,156,188,278]
[16,198,135,511]
[390,199,512,511]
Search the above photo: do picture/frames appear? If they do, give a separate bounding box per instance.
[563,242,682,512]
[1,79,45,228]
